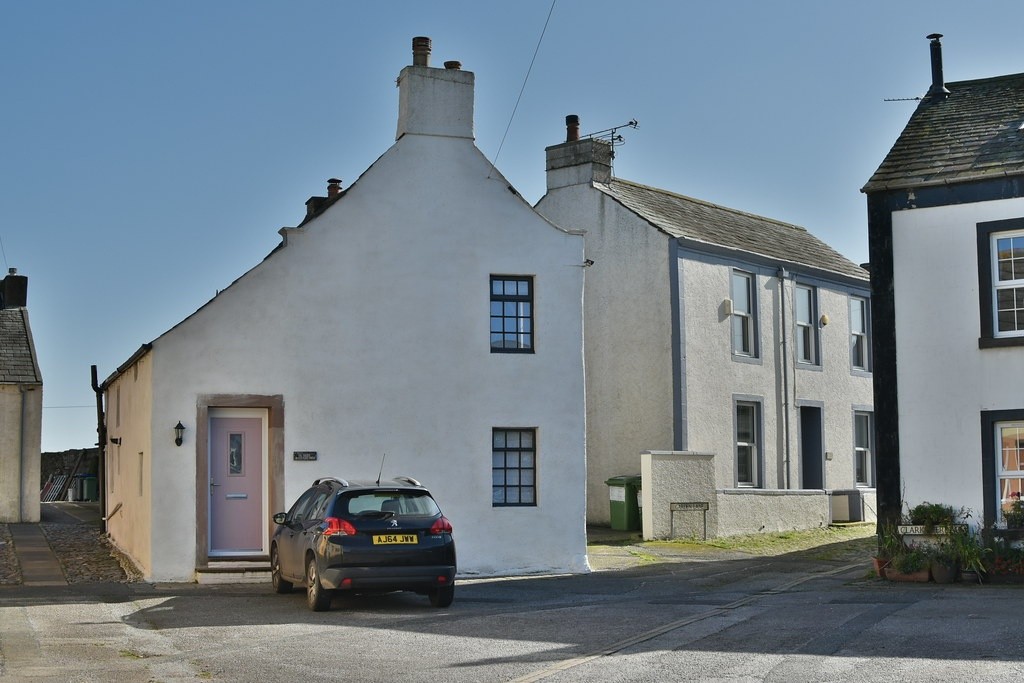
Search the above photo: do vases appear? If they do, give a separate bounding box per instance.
[989,573,1022,585]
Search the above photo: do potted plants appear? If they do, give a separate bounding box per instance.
[869,520,894,574]
[883,544,931,583]
[954,526,993,587]
[925,540,961,585]
[905,500,964,536]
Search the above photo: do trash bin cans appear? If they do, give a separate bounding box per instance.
[76,473,96,501]
[79,477,98,502]
[605,475,638,531]
[631,476,643,531]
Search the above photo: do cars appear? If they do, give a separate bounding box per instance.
[269,453,457,611]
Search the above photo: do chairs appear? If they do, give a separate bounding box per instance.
[381,499,400,515]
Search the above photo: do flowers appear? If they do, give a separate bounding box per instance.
[981,552,1024,576]
[1002,489,1024,517]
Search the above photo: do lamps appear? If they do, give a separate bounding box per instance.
[174,419,185,447]
[821,314,830,326]
